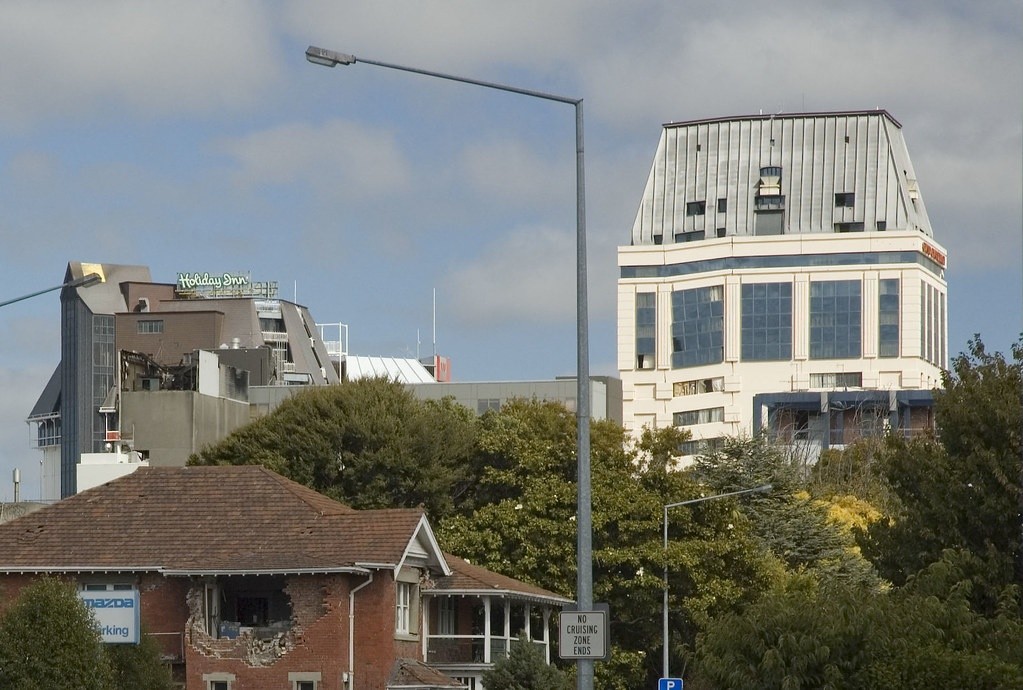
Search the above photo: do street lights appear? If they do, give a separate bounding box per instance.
[662,484,773,679]
[306,45,593,690]
